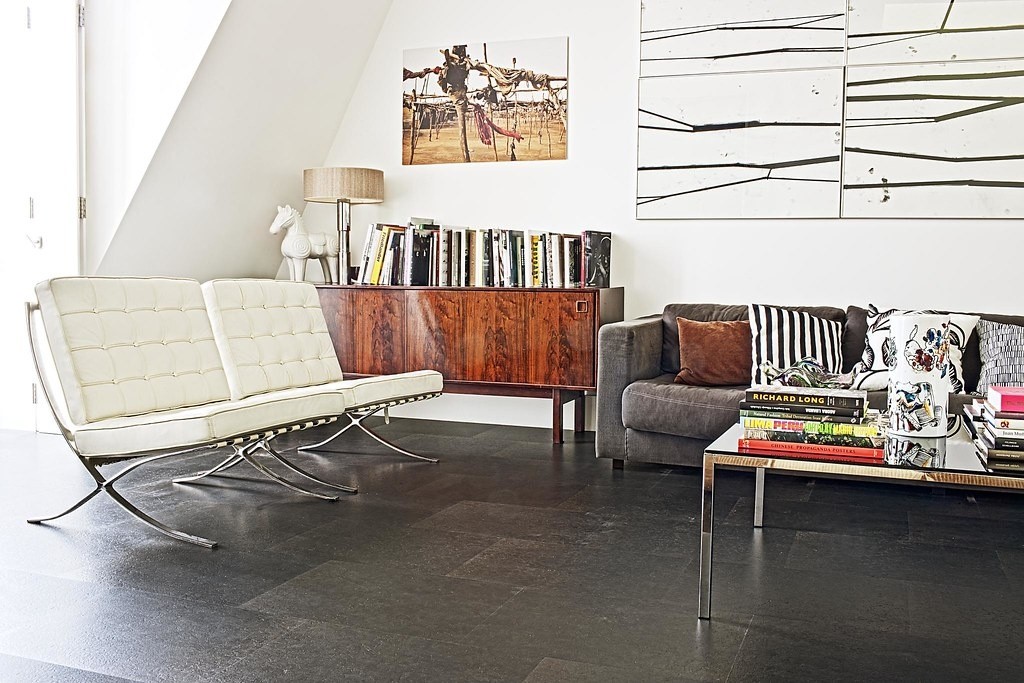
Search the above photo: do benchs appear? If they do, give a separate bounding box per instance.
[23,277,445,550]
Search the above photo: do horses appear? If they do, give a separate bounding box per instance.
[270,205,339,286]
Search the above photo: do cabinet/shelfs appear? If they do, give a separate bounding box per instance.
[313,281,624,445]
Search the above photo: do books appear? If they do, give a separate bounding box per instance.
[738,384,886,465]
[357,217,612,289]
[960,385,1024,475]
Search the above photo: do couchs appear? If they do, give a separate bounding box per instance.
[594,304,1024,496]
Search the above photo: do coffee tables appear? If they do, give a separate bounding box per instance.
[697,409,1024,620]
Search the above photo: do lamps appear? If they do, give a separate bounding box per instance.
[302,167,385,285]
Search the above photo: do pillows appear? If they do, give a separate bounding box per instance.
[975,319,1024,394]
[849,303,981,394]
[673,316,753,386]
[747,303,844,388]
